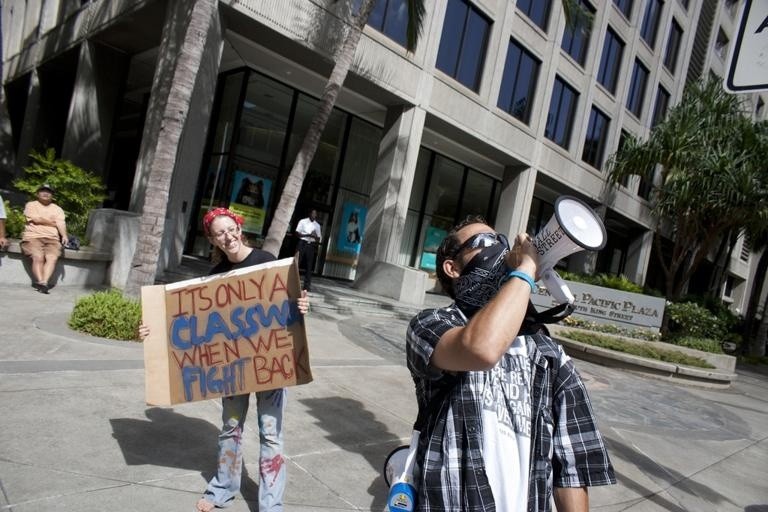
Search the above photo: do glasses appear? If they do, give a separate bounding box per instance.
[452,233,510,259]
[211,226,237,237]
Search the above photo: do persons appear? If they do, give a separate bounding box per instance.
[406,216,617,512]
[296,209,322,292]
[138,207,310,512]
[0,195,10,249]
[19,187,69,294]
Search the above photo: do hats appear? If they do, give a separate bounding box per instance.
[37,184,54,192]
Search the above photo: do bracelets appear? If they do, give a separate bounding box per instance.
[498,271,538,294]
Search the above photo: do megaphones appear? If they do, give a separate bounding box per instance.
[532,195,608,305]
